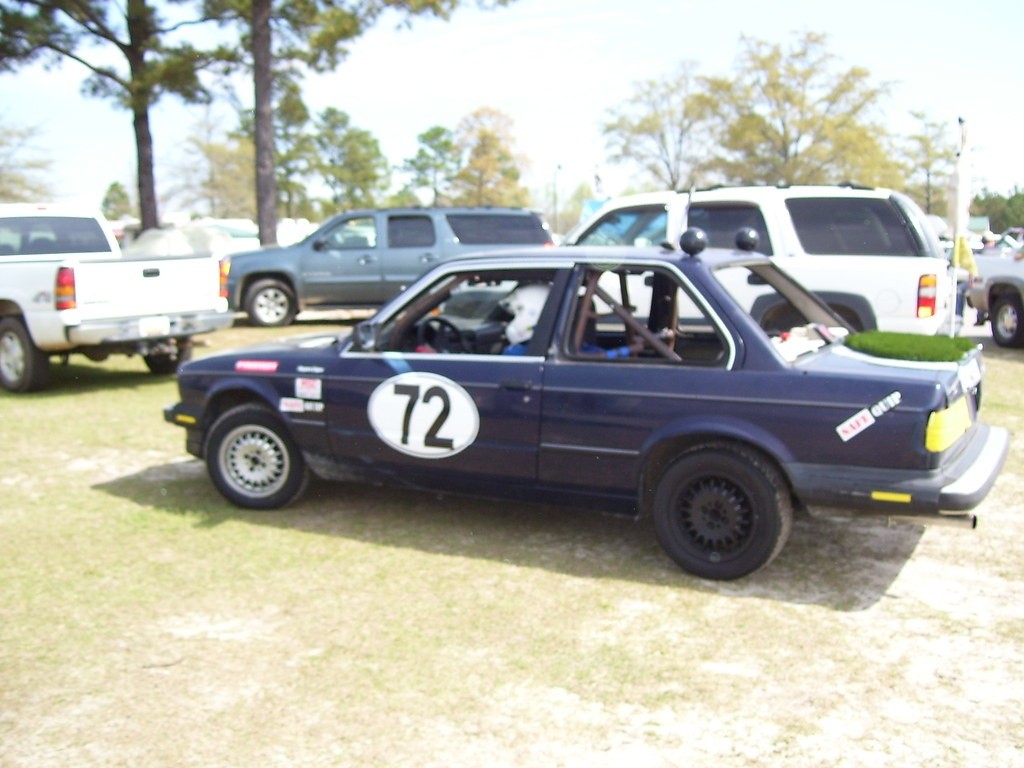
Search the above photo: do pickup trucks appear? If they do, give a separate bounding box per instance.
[0,202,235,390]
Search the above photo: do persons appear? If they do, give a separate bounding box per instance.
[417,277,553,355]
[973,230,1002,326]
[951,236,984,326]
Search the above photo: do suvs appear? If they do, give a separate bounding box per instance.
[220,206,568,327]
[505,182,949,344]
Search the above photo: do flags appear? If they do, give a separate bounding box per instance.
[946,126,972,237]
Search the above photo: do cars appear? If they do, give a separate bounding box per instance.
[161,227,1007,583]
[970,238,1024,350]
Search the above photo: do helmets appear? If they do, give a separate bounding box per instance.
[499,281,553,345]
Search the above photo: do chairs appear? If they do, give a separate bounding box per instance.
[565,296,598,351]
[27,238,57,253]
